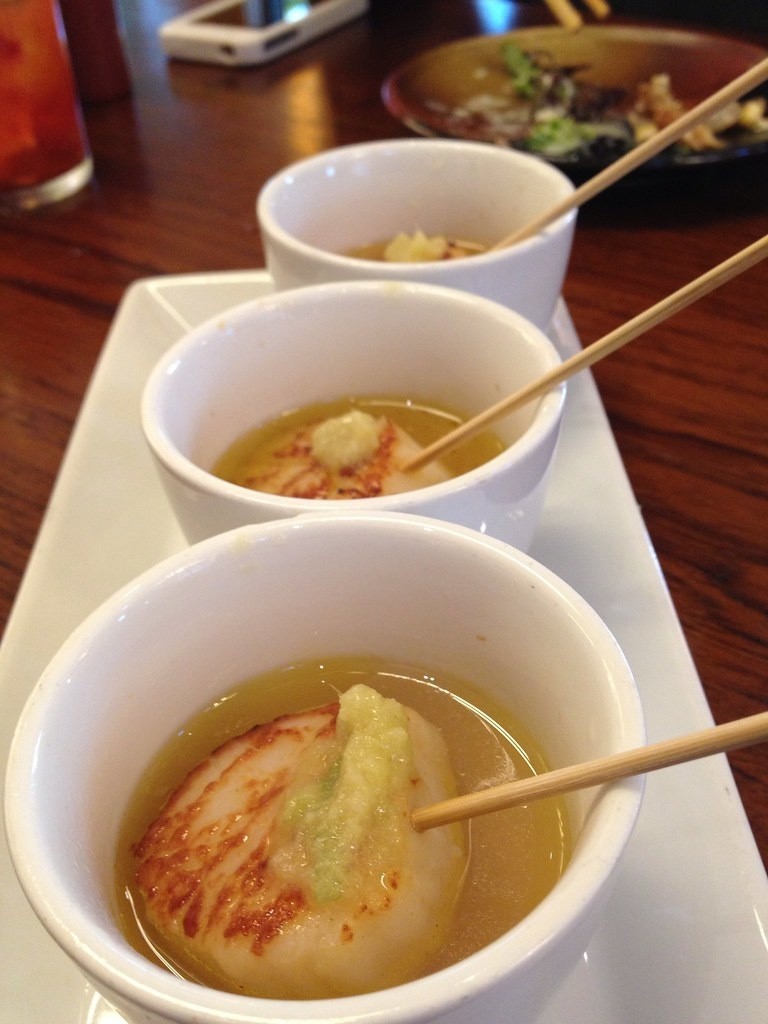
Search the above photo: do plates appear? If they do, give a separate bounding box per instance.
[381,24,768,166]
[0,270,768,1024]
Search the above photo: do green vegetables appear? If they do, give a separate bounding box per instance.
[503,42,562,103]
[527,116,595,154]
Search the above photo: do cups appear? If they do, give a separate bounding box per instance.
[0,0,93,210]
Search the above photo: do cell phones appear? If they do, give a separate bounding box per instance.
[158,0,371,66]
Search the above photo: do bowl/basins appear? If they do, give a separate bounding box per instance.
[140,281,566,550]
[256,136,577,331]
[4,511,647,1024]
